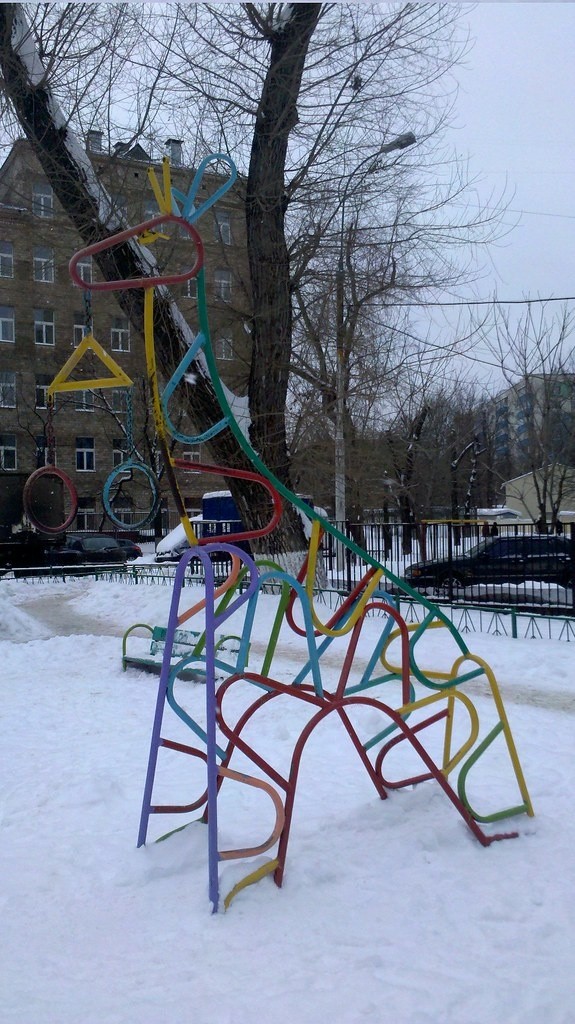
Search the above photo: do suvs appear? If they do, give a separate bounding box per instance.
[403,532,575,596]
[116,539,143,561]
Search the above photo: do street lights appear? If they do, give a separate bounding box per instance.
[337,130,417,594]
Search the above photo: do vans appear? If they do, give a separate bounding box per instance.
[156,514,204,562]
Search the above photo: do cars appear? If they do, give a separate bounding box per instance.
[14,533,126,579]
